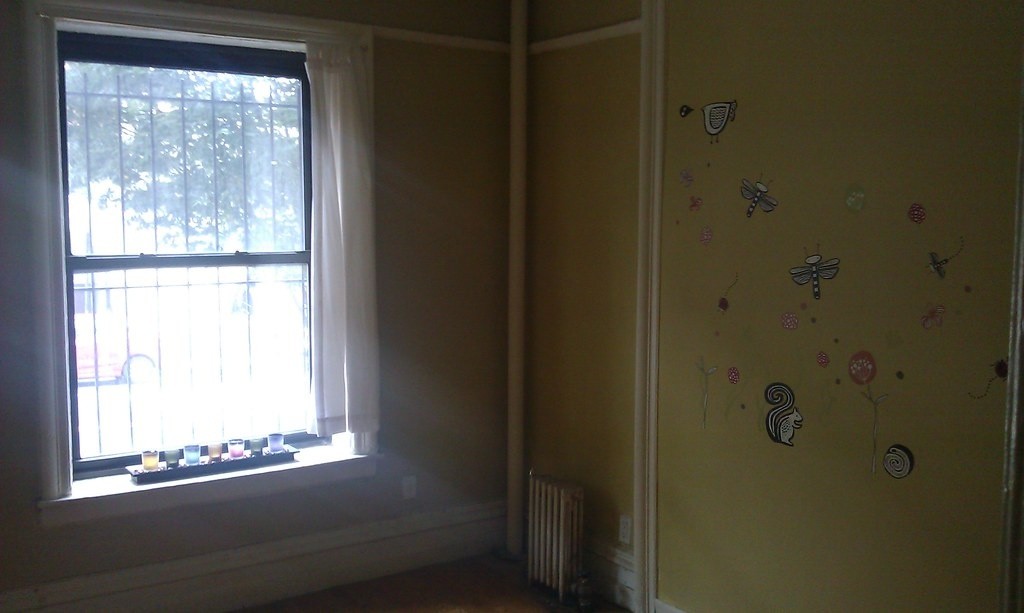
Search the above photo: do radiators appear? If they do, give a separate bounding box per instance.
[524,468,591,608]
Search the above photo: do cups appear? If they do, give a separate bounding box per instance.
[208,443,222,464]
[267,434,284,452]
[250,439,263,457]
[184,445,201,466]
[142,450,159,470]
[165,450,180,470]
[228,438,244,459]
[332,432,354,459]
